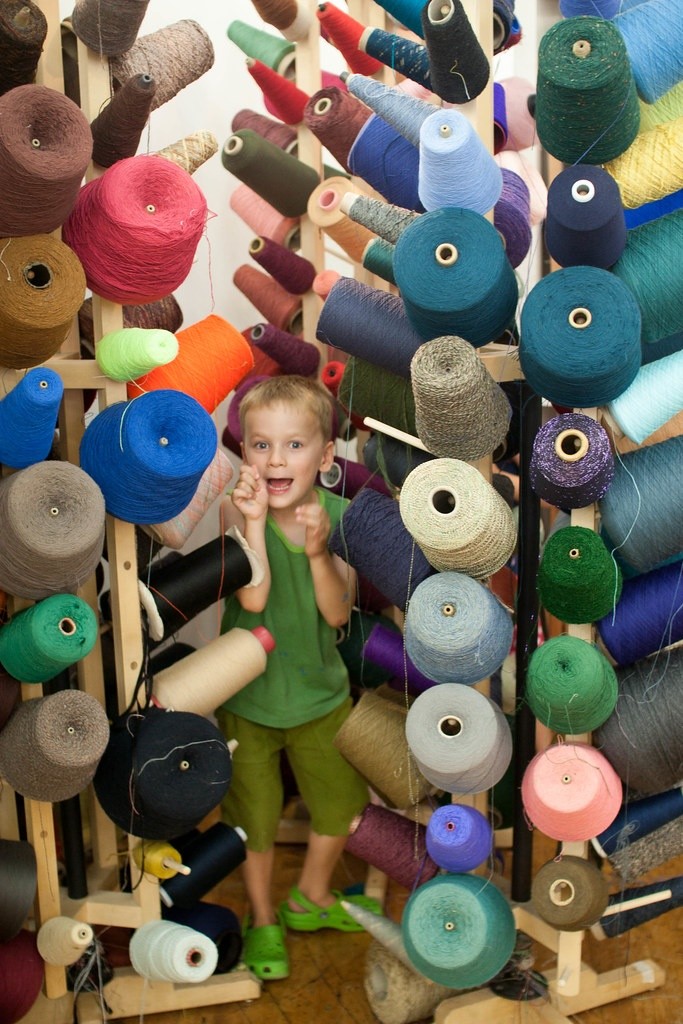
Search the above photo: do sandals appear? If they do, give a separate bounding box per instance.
[278,885,383,932]
[242,906,289,980]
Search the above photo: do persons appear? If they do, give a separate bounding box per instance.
[215,375,384,980]
